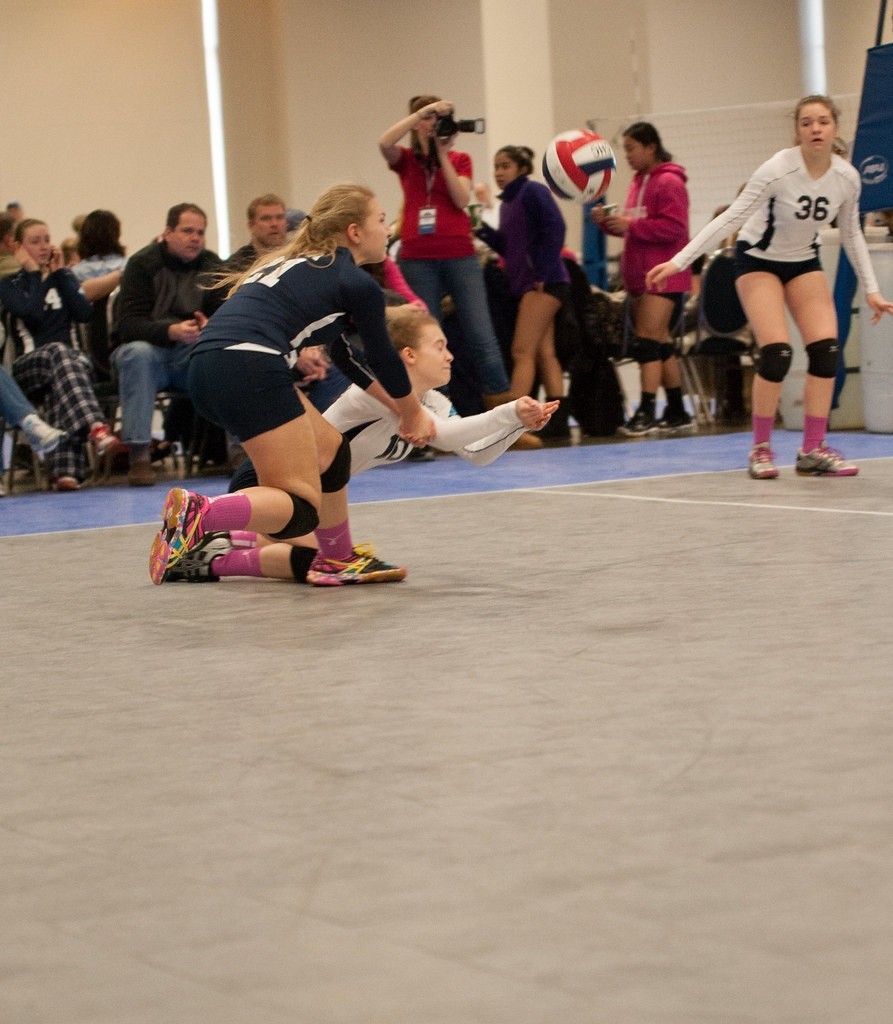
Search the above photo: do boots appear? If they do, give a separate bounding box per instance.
[531,397,572,440]
[484,392,545,450]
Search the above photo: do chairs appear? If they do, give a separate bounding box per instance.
[587,283,759,429]
[0,285,224,498]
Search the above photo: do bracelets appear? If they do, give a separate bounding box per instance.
[415,112,422,119]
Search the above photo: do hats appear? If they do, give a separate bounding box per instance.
[285,207,306,230]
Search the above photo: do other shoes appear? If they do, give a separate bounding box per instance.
[11,443,34,470]
[714,398,736,426]
[86,442,100,475]
[127,459,156,485]
[405,448,434,460]
[228,444,248,475]
[116,438,178,474]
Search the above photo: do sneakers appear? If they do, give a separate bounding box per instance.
[57,477,81,489]
[652,406,693,432]
[87,424,121,456]
[306,542,408,585]
[163,530,233,583]
[748,442,779,479]
[618,408,658,436]
[26,422,70,453]
[149,488,212,586]
[796,440,859,476]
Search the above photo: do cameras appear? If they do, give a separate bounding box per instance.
[433,113,486,139]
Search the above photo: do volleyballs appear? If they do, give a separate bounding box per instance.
[541,127,616,204]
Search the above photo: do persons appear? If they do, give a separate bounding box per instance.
[379,95,543,450]
[164,304,560,579]
[472,145,573,438]
[692,204,745,423]
[150,183,408,585]
[0,212,126,492]
[496,248,593,369]
[591,121,692,436]
[358,255,428,322]
[7,202,23,219]
[108,202,249,486]
[645,90,893,479]
[223,192,290,274]
